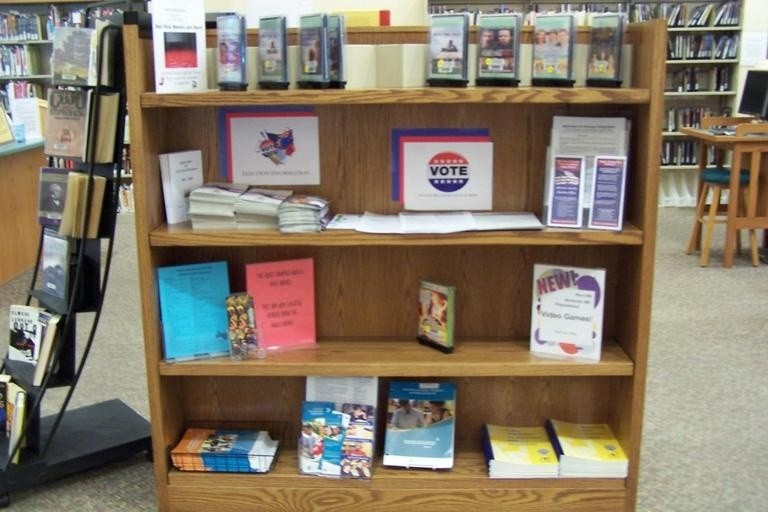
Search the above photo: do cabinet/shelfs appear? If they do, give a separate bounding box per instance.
[1,1,746,512]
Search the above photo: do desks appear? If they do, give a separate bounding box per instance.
[680,115,768,266]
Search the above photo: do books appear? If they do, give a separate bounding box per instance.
[655,2,740,166]
[1,4,119,135]
[429,2,652,32]
[168,376,631,480]
[158,148,329,236]
[1,90,120,463]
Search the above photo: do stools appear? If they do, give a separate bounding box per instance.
[687,167,760,267]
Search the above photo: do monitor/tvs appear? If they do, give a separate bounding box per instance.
[736,69,768,121]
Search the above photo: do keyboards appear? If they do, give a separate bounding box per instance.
[709,127,736,136]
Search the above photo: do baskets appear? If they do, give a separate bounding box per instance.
[166,420,288,473]
